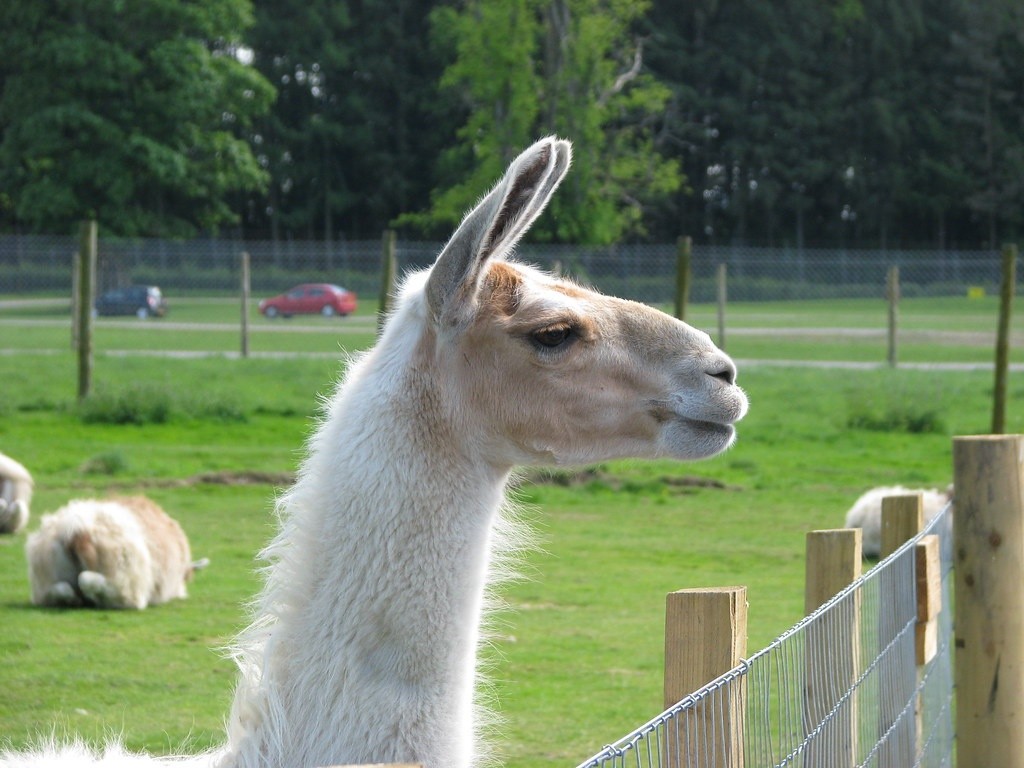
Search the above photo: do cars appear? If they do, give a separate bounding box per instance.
[93,285,167,320]
[257,284,357,319]
